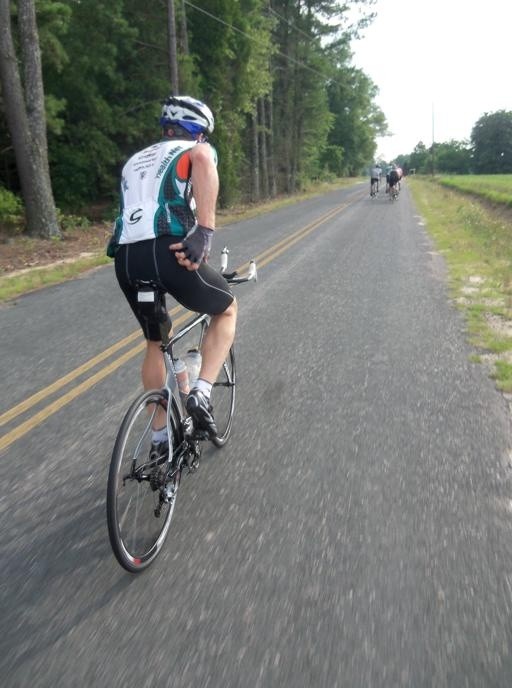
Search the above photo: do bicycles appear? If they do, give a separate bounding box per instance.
[371,182,380,198]
[386,181,401,200]
[106,246,257,573]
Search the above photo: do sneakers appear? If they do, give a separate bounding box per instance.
[147,439,169,462]
[185,387,218,438]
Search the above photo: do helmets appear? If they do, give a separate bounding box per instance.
[160,95,216,135]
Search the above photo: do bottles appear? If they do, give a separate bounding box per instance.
[173,349,202,395]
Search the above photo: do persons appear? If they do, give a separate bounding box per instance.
[107,94,238,466]
[370,162,402,196]
